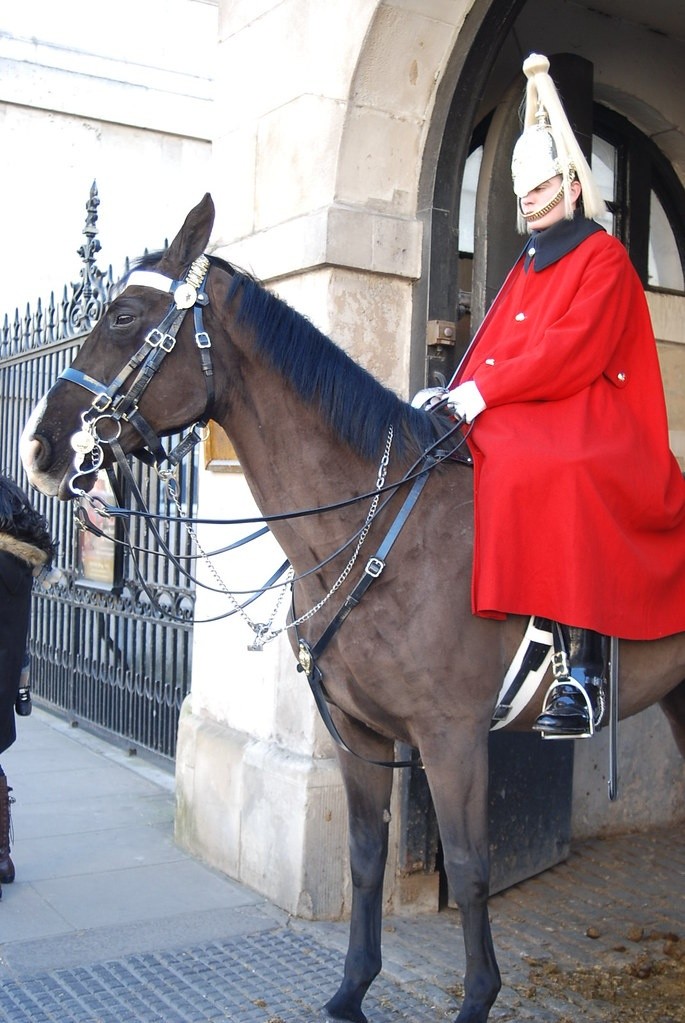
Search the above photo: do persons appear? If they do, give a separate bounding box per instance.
[0,468,60,899]
[411,53,685,735]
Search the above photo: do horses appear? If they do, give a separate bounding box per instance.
[17,191,684,1023]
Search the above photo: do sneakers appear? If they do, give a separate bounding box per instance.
[15,686,32,715]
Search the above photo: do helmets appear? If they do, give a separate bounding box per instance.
[511,53,604,235]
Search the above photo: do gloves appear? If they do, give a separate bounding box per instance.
[441,381,486,424]
[411,387,449,411]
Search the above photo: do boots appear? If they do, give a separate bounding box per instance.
[531,621,603,735]
[0,776,16,883]
